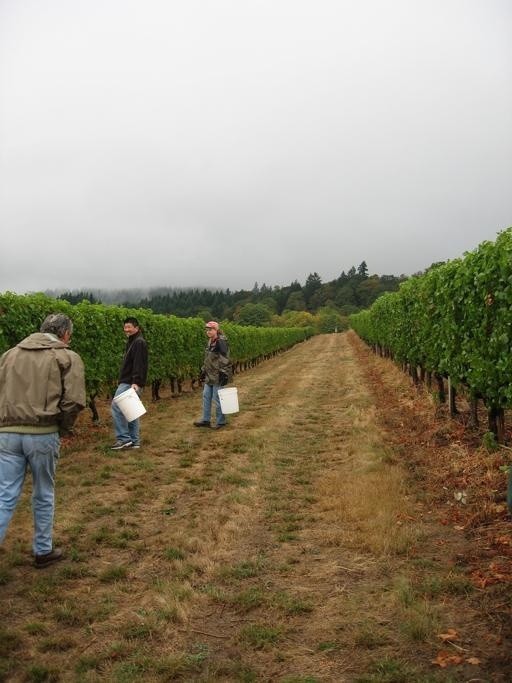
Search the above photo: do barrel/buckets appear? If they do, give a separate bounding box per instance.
[218,386,240,415]
[112,386,148,423]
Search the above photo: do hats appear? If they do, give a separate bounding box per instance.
[206,321,218,328]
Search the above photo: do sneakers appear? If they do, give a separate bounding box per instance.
[193,421,209,427]
[34,549,62,568]
[111,440,140,450]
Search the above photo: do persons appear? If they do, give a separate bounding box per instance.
[193,320,233,429]
[110,315,149,450]
[0,312,87,566]
